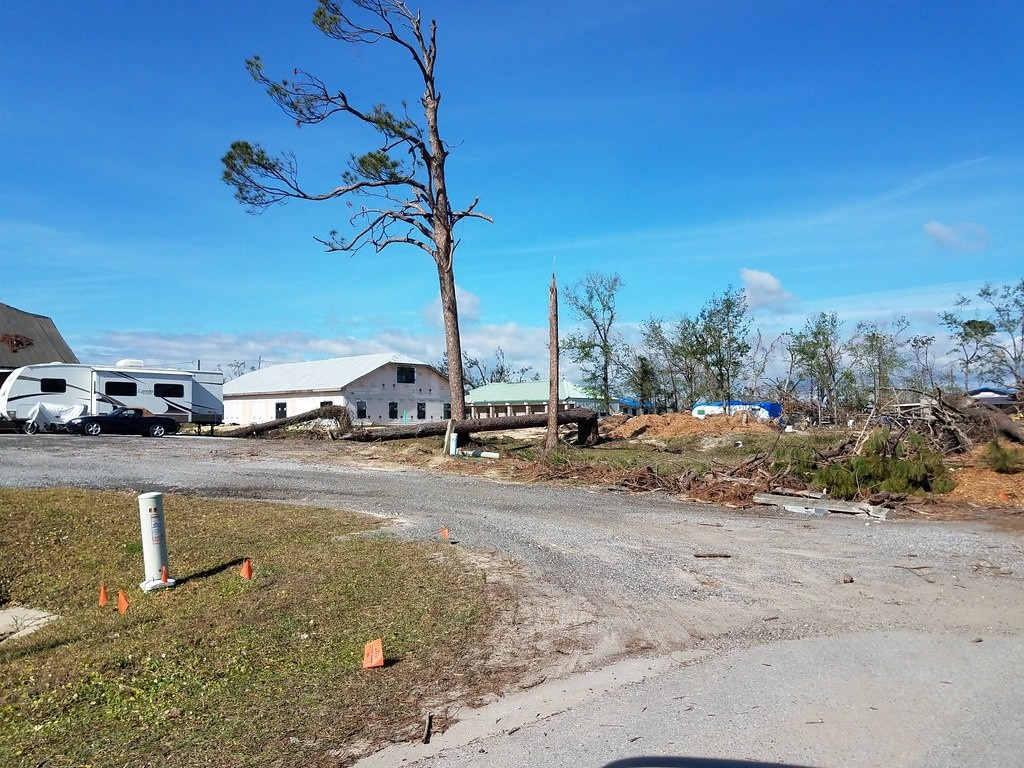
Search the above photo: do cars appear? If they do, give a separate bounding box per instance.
[66,406,181,437]
